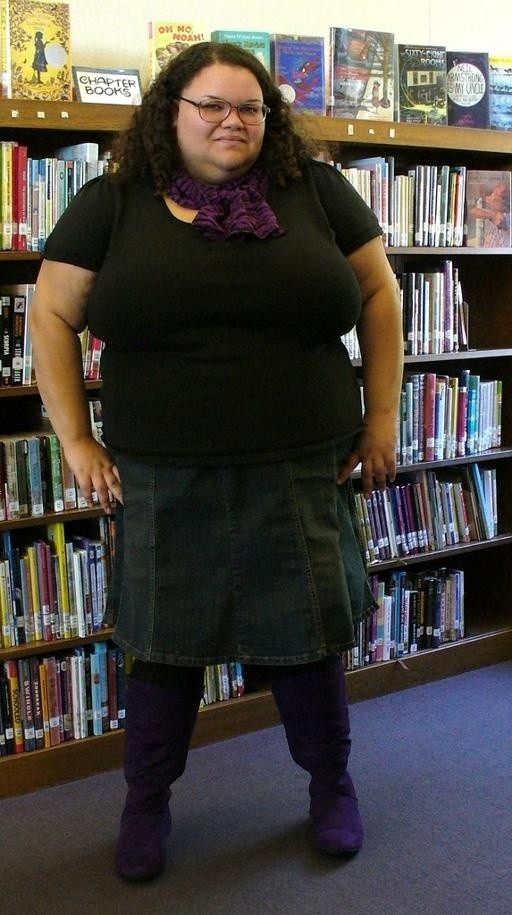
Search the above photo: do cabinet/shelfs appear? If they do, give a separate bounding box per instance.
[0,101,512,804]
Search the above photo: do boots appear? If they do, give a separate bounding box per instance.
[114,674,205,881]
[274,663,363,854]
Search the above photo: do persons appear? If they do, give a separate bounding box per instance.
[29,42,405,885]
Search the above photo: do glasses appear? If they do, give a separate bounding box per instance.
[179,96,270,125]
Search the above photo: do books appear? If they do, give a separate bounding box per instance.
[274,36,326,117]
[149,23,204,87]
[1,141,511,755]
[390,44,447,127]
[2,1,72,103]
[489,56,512,127]
[445,51,489,126]
[326,29,398,121]
[212,31,271,81]
